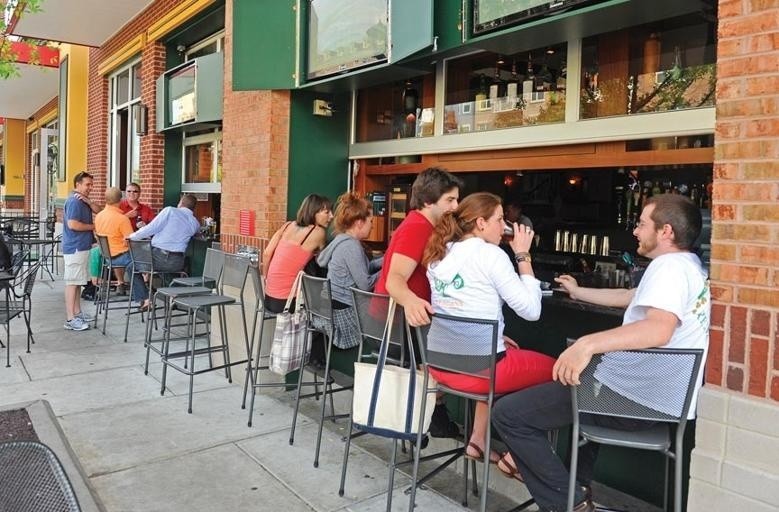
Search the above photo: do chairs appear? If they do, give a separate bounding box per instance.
[237,249,319,428]
[94,233,145,335]
[408,313,552,512]
[288,270,405,470]
[8,261,44,345]
[165,241,221,332]
[7,249,33,309]
[553,329,704,512]
[0,280,35,368]
[144,247,229,397]
[0,216,39,240]
[0,437,87,512]
[124,239,190,348]
[338,284,478,512]
[41,232,63,282]
[160,252,254,414]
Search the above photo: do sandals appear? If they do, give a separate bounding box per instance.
[495,454,524,484]
[464,442,502,464]
[139,301,153,311]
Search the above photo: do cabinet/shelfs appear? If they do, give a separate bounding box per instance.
[156,51,224,135]
[460,1,716,56]
[231,1,435,92]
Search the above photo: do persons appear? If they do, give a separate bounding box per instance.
[420,192,557,483]
[61,171,102,330]
[363,166,463,448]
[80,246,103,302]
[94,187,148,286]
[491,191,710,512]
[114,182,154,296]
[501,200,533,246]
[315,192,384,351]
[126,194,201,312]
[261,193,333,373]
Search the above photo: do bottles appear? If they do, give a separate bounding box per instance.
[474,73,489,117]
[612,174,715,231]
[536,52,552,104]
[521,52,537,109]
[488,58,504,112]
[556,57,568,98]
[506,56,519,110]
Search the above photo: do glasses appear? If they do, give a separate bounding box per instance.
[80,169,87,176]
[127,188,138,195]
[637,220,656,232]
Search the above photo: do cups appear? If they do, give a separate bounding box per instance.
[608,269,625,287]
[599,235,609,257]
[503,219,520,241]
[570,233,577,252]
[554,231,561,251]
[578,235,587,254]
[588,236,597,255]
[561,231,569,252]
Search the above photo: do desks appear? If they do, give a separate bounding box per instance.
[5,239,62,268]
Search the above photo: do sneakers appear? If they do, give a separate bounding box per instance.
[77,313,95,322]
[64,318,90,331]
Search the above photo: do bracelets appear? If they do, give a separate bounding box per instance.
[514,252,532,264]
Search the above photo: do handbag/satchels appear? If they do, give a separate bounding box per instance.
[268,271,311,375]
[353,295,437,442]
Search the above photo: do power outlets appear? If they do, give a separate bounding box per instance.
[313,99,335,117]
